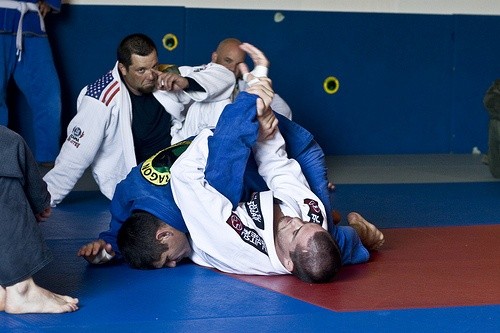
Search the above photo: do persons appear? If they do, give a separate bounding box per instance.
[0,0,60,176]
[0,125,78,315]
[43,34,386,283]
